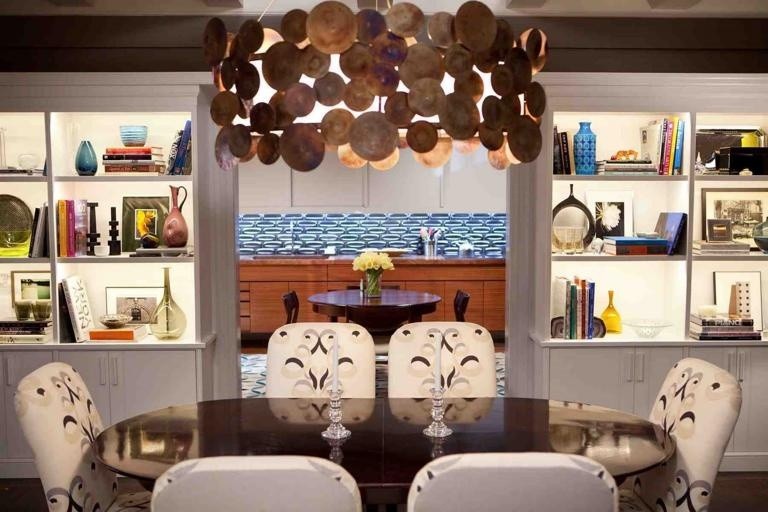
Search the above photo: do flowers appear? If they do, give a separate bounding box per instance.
[596,203,622,233]
[351,248,393,271]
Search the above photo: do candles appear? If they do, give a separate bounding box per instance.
[331,333,340,392]
[435,333,441,392]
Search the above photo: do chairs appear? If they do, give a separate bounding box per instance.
[15,359,153,511]
[267,319,378,401]
[151,452,365,512]
[451,287,470,322]
[386,316,496,400]
[407,451,622,512]
[281,291,300,325]
[611,356,740,512]
[343,304,406,332]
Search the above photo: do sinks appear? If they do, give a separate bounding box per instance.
[252,255,328,260]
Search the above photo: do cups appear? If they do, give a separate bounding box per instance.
[551,226,586,255]
[13,301,32,321]
[16,152,41,169]
[31,300,52,320]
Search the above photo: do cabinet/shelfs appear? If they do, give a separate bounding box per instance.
[484,259,507,332]
[506,72,768,340]
[505,345,766,475]
[326,259,405,328]
[0,71,216,349]
[405,260,484,327]
[239,260,328,333]
[0,346,218,481]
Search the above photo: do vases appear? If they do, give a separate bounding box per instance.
[598,285,621,333]
[150,267,186,342]
[365,269,382,299]
[574,120,597,175]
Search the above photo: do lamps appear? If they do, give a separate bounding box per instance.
[205,0,546,170]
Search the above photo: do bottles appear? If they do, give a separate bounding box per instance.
[359,292,364,299]
[598,288,623,332]
[360,279,364,291]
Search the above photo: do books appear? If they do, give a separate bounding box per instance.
[553,126,576,175]
[58,275,146,343]
[58,199,89,258]
[603,213,687,255]
[29,202,51,258]
[690,313,761,340]
[552,277,596,339]
[164,120,192,175]
[1,317,54,343]
[596,118,683,175]
[102,147,166,176]
[693,240,750,255]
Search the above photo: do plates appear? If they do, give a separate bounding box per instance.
[0,192,34,249]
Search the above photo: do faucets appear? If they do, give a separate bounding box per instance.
[290,221,294,254]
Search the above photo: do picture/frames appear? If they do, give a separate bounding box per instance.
[699,188,767,251]
[9,269,52,309]
[587,191,633,242]
[706,217,732,244]
[120,196,169,257]
[104,286,169,336]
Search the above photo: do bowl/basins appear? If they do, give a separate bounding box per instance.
[622,314,670,337]
[119,123,147,147]
[753,236,767,254]
[100,314,132,328]
